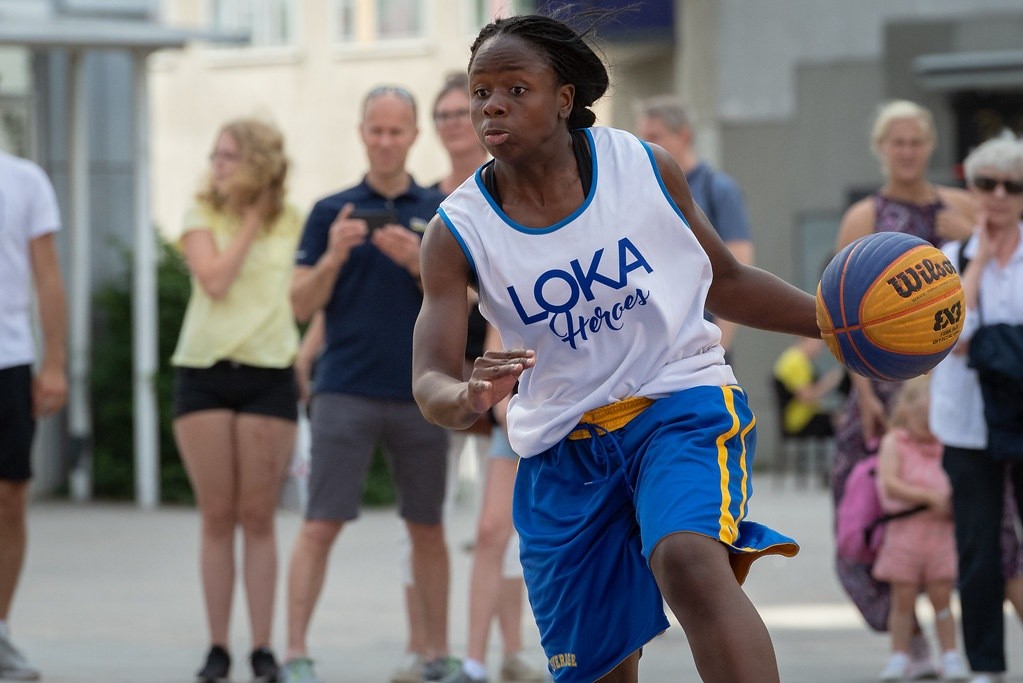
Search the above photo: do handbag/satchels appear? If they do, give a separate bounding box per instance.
[838,454,886,561]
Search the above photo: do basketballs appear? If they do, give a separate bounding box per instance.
[815,228,968,383]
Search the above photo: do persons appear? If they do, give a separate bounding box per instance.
[170,120,324,682]
[391,72,549,682]
[838,101,1023,683]
[0,146,68,683]
[634,98,753,352]
[875,376,970,683]
[283,87,464,683]
[770,338,839,438]
[411,15,822,682]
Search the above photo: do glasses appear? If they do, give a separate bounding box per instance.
[974,177,1022,194]
[432,108,471,125]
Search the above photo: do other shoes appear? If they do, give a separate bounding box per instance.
[198,647,231,683]
[281,657,318,683]
[249,649,280,683]
[0,636,41,683]
[878,658,913,683]
[422,659,476,682]
[938,653,970,682]
[390,655,420,681]
[504,651,549,683]
